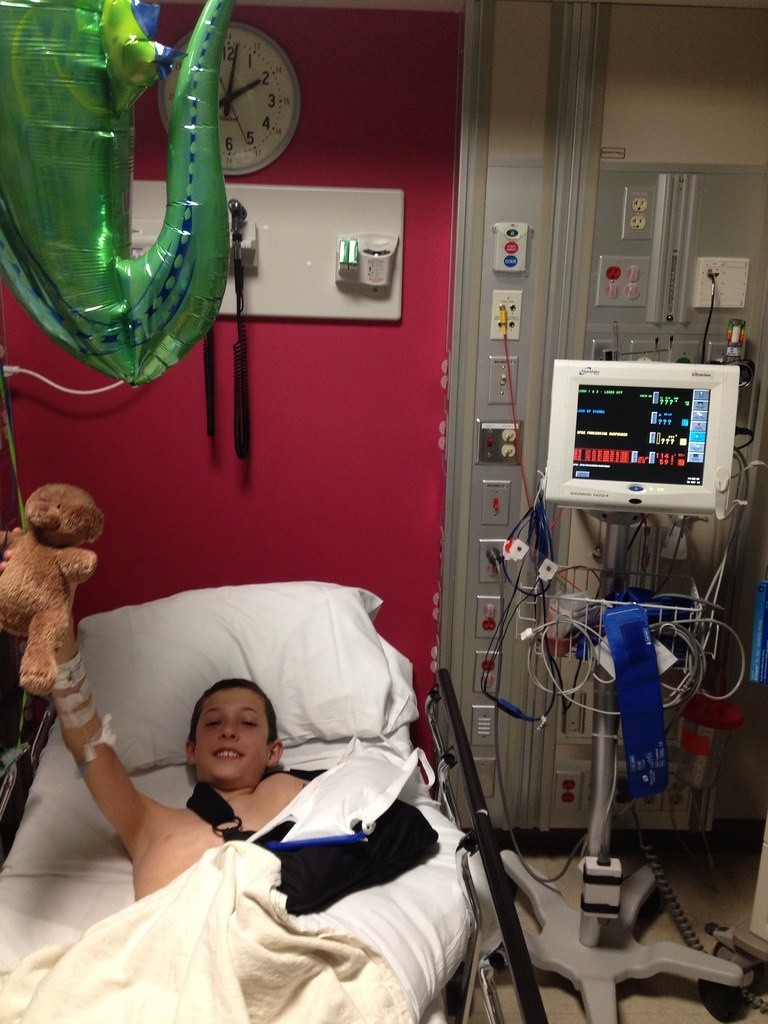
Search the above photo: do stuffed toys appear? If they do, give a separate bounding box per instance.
[0,481,107,699]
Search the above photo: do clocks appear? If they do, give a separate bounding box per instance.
[156,21,302,175]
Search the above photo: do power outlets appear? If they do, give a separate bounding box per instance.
[622,184,655,240]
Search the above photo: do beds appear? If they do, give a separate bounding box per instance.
[0,634,549,1023]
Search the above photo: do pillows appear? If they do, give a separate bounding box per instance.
[76,582,420,775]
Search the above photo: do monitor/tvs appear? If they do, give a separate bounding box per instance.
[547,359,740,519]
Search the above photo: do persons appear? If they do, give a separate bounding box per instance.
[0,526,442,913]
